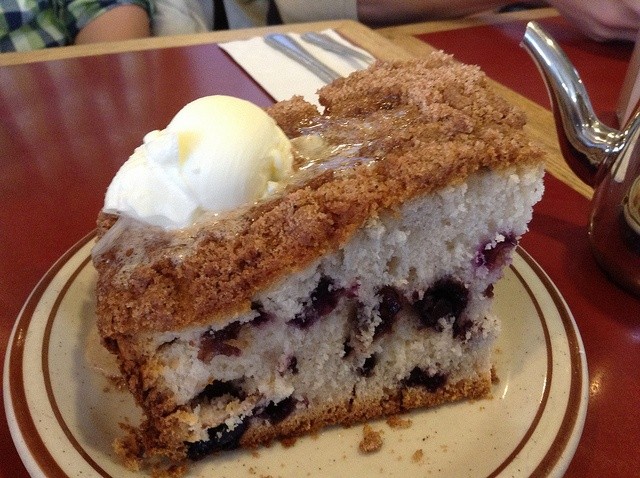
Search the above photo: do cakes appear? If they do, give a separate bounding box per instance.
[91,48,549,478]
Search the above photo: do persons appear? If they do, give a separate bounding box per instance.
[1,0,148,52]
[150,0,281,31]
[278,1,640,43]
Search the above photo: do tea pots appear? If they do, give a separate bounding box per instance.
[507,17,640,300]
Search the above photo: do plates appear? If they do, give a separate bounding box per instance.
[0,214,590,474]
